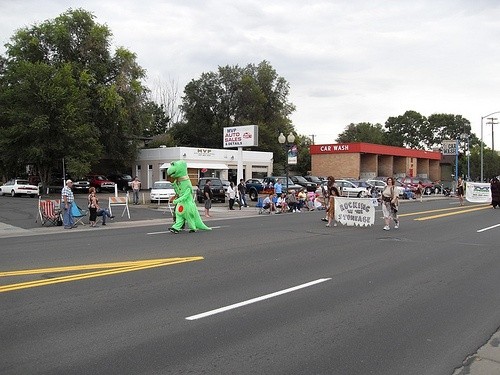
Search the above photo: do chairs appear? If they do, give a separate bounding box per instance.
[272,198,284,212]
[35,197,104,225]
[257,198,271,215]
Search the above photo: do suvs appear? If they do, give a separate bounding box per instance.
[106,174,142,192]
[315,180,369,199]
[65,174,91,194]
[87,174,116,193]
[195,178,226,204]
[290,176,321,192]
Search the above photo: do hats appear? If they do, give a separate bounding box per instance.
[66,180,73,183]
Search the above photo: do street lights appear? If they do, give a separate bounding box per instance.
[278,132,295,195]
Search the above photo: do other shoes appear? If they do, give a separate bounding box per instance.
[321,217,328,222]
[325,224,331,226]
[293,209,301,213]
[102,222,106,225]
[90,224,99,227]
[275,211,281,214]
[64,225,77,229]
[110,216,115,218]
[383,225,390,230]
[133,202,140,205]
[394,221,399,228]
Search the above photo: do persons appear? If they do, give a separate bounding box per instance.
[326,187,342,227]
[227,181,236,210]
[358,186,383,203]
[321,176,340,222]
[416,181,424,202]
[381,177,400,230]
[490,176,500,209]
[457,176,465,206]
[204,179,213,217]
[238,179,249,209]
[87,187,99,227]
[60,179,78,229]
[95,198,115,225]
[260,187,326,214]
[131,176,140,205]
[273,178,283,198]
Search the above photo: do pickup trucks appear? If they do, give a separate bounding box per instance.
[245,177,307,202]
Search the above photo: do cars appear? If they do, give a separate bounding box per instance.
[149,180,176,203]
[245,178,263,184]
[352,177,442,199]
[0,179,40,199]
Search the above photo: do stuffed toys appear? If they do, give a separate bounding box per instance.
[167,160,212,234]
